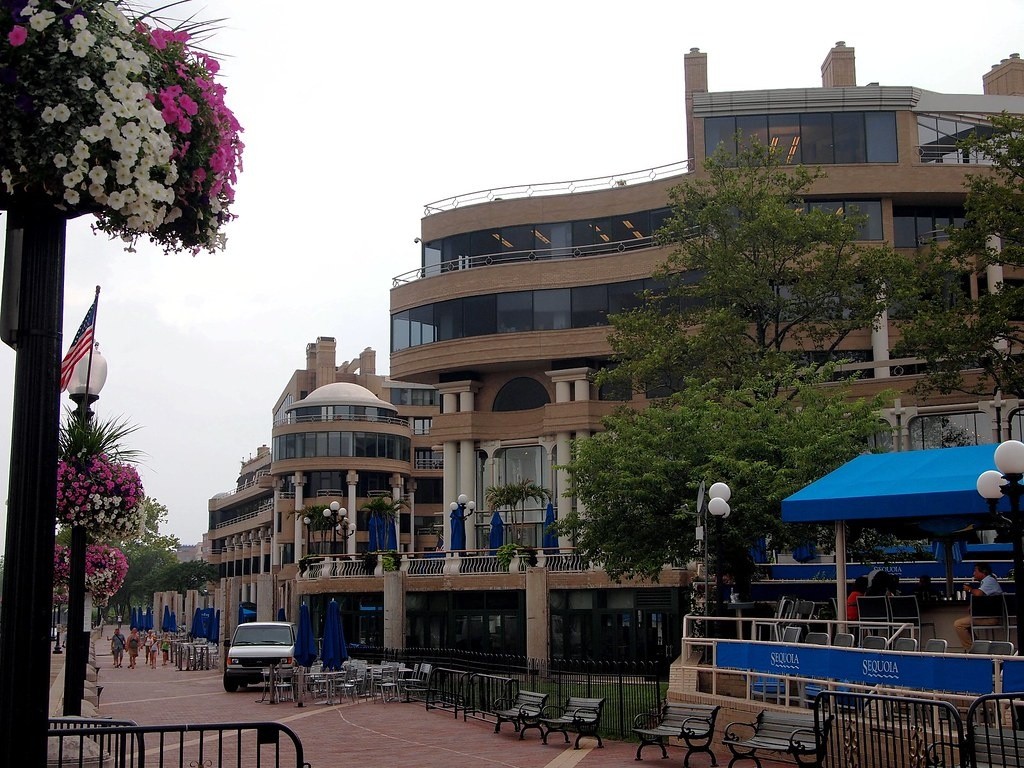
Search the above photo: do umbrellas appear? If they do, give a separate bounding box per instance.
[450,504,463,549]
[369,512,397,550]
[543,504,561,555]
[191,607,204,639]
[792,544,816,564]
[130,606,154,633]
[294,601,316,707]
[751,536,769,564]
[162,604,176,636]
[316,598,348,704]
[211,608,220,645]
[490,512,503,555]
[238,605,245,624]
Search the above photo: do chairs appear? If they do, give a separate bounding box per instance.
[256,660,431,707]
[756,593,1019,729]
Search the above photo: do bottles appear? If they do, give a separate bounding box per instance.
[730,587,735,603]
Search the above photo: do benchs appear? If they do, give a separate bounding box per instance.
[492,689,549,741]
[538,696,606,750]
[631,700,721,768]
[722,709,834,768]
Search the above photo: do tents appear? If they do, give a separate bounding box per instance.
[781,444,1023,633]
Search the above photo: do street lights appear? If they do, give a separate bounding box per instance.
[708,481,731,639]
[336,516,356,556]
[63,343,108,717]
[450,494,477,557]
[975,441,1024,732]
[324,501,347,576]
[304,517,312,555]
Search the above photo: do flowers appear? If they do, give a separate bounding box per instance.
[0,0,245,257]
[53,402,158,609]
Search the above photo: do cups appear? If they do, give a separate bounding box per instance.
[964,583,969,586]
[962,591,967,600]
[956,591,962,600]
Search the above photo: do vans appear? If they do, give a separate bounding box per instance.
[223,621,299,693]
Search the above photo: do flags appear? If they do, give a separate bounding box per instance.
[61,297,98,392]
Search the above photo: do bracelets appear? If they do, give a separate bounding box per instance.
[968,588,972,592]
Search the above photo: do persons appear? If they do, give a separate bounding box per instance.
[92,621,97,629]
[847,576,868,645]
[60,626,67,648]
[952,563,1003,649]
[116,613,123,629]
[107,628,171,669]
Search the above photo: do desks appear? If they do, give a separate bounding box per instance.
[379,668,413,702]
[359,666,393,697]
[309,672,347,704]
